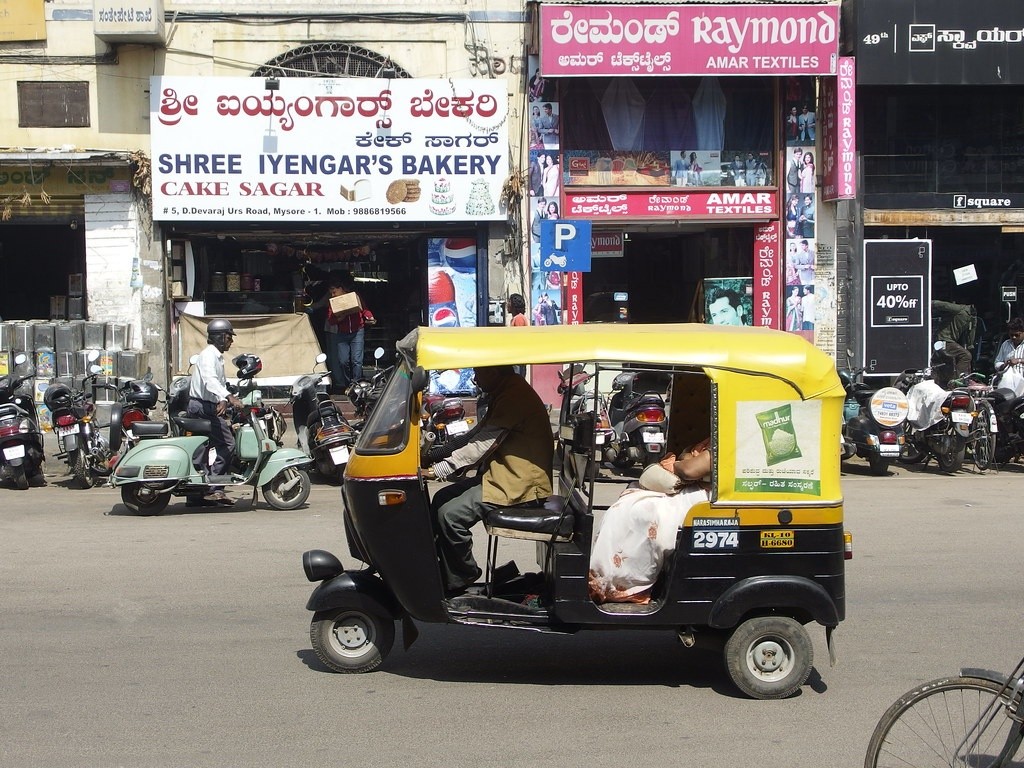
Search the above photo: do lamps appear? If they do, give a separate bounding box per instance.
[264,69,279,98]
[374,55,395,79]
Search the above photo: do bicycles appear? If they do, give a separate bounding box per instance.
[864,653,1024,768]
[947,370,1000,474]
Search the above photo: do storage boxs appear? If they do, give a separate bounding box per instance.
[175,301,206,318]
[329,292,363,317]
[0,274,151,434]
[172,245,185,296]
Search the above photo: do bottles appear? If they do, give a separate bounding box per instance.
[211,271,261,293]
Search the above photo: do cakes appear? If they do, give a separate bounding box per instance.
[430,178,495,215]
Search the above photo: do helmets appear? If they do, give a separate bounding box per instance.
[45,383,73,411]
[207,318,237,336]
[126,379,157,408]
[231,353,263,378]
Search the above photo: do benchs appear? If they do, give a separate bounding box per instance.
[483,494,573,544]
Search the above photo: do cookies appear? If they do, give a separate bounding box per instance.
[386,179,421,204]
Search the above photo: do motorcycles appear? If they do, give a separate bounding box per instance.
[420,369,467,442]
[337,345,435,470]
[108,400,312,514]
[604,365,669,466]
[43,349,109,488]
[0,354,45,488]
[556,360,614,468]
[834,348,910,472]
[298,321,852,695]
[222,352,288,444]
[975,359,1024,467]
[153,353,198,438]
[91,366,160,451]
[277,351,356,482]
[888,339,976,473]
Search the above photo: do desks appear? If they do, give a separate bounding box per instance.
[204,291,296,314]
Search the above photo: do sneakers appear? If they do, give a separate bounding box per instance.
[210,490,235,506]
[186,495,219,507]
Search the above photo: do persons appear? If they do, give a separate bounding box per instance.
[994,317,1024,464]
[528,57,817,333]
[326,277,377,387]
[420,365,554,597]
[931,300,979,387]
[187,319,245,505]
[507,294,528,379]
[589,436,711,603]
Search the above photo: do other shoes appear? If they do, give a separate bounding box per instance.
[443,567,482,599]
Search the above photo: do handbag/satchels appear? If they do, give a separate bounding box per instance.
[639,460,686,497]
